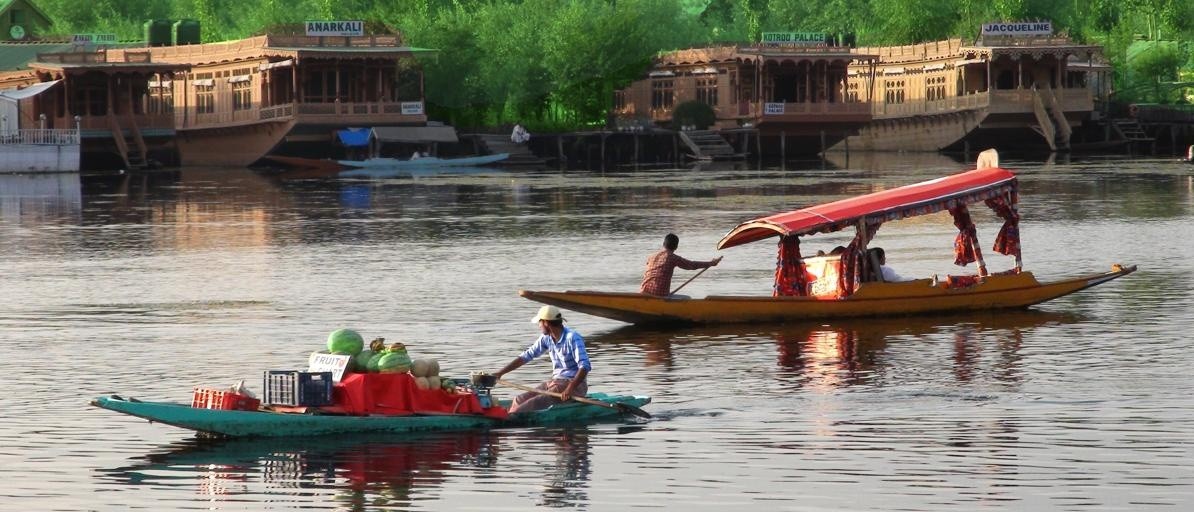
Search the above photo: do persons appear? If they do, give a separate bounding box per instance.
[873,248,914,282]
[640,233,721,296]
[491,304,591,413]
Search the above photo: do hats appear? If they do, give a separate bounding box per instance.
[532,305,562,323]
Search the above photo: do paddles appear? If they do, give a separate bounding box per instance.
[491,376,651,419]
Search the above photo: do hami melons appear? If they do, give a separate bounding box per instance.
[411,359,442,390]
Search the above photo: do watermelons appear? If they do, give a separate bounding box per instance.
[327,329,411,373]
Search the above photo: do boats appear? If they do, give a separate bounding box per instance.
[91,392,651,439]
[517,149,1137,325]
[338,153,509,169]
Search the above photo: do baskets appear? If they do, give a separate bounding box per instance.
[191,389,260,411]
[264,370,333,407]
